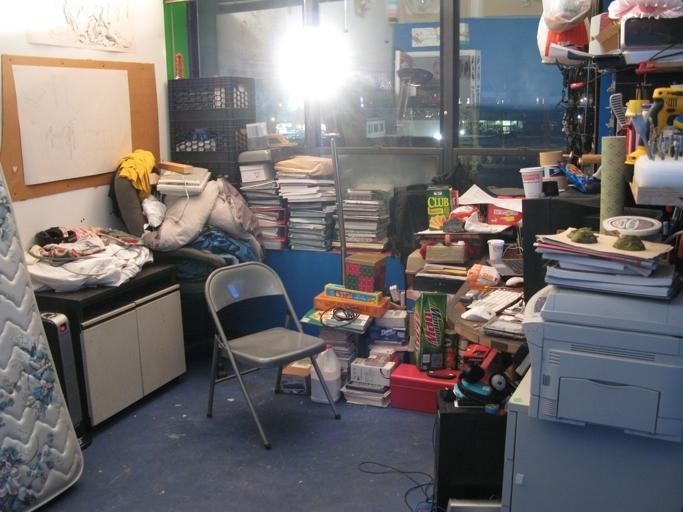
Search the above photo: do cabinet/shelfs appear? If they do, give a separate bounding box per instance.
[31,258,188,431]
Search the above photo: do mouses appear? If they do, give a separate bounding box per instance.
[460,305,497,322]
[506,277,524,287]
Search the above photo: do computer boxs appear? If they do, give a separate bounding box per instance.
[432,389,508,512]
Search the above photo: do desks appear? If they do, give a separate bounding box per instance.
[444,247,530,359]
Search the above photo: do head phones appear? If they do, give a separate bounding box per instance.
[35,227,77,248]
[456,364,511,404]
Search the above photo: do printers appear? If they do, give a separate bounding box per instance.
[522,285,683,444]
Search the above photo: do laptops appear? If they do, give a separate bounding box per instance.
[486,259,524,279]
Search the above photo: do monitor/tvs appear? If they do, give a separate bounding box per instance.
[522,194,664,308]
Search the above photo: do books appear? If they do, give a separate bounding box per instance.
[239,171,390,252]
[301,307,392,409]
[532,227,677,300]
[414,227,487,275]
[98,228,143,247]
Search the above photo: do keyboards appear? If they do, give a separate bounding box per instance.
[465,289,522,315]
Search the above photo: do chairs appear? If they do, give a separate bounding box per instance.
[114,160,242,353]
[204,261,341,445]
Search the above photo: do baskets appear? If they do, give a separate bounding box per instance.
[168,76,256,198]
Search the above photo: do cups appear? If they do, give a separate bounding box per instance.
[487,238,505,261]
[519,167,544,199]
[539,150,563,167]
[541,164,568,192]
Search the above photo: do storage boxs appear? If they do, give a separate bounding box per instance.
[344,254,390,293]
[351,290,462,416]
